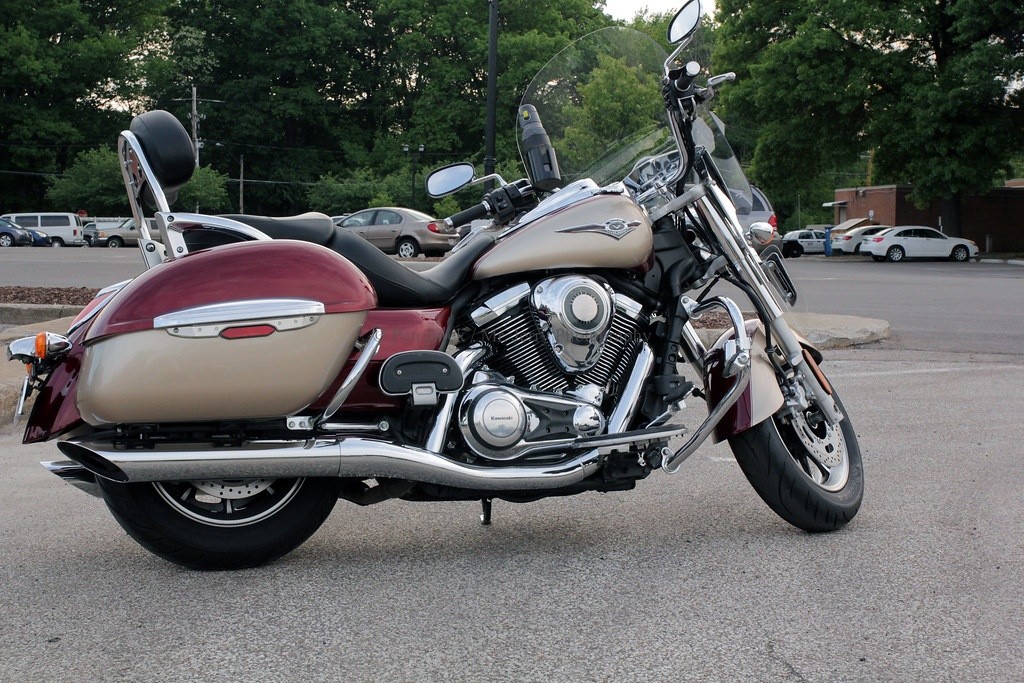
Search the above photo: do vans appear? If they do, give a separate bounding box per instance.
[664,173,780,253]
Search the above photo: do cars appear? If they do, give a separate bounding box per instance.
[859,224,979,263]
[830,225,893,256]
[334,206,462,259]
[783,230,832,255]
[0,211,165,248]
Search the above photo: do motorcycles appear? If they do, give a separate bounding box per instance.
[6,0,869,572]
